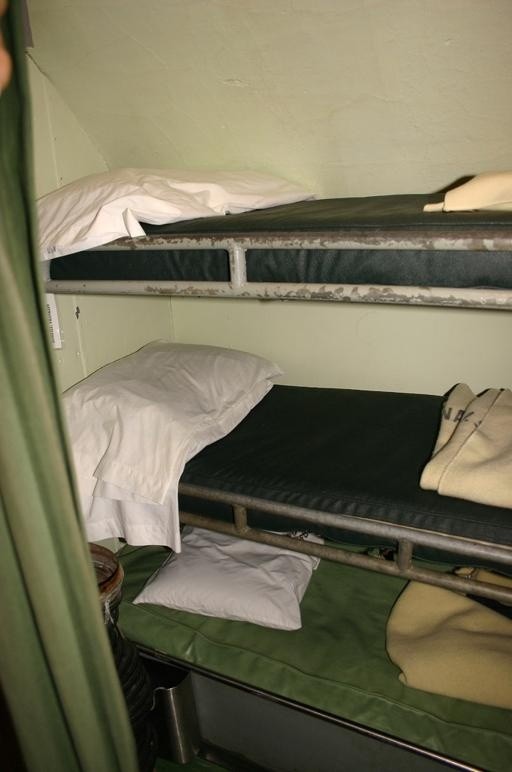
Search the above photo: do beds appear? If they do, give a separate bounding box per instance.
[30,177,511,772]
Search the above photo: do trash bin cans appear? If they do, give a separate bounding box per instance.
[142,658,202,765]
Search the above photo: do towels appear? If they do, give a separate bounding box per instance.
[418,378,511,511]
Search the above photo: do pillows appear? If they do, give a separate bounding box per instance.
[134,518,327,630]
[60,338,284,467]
[34,168,320,269]
